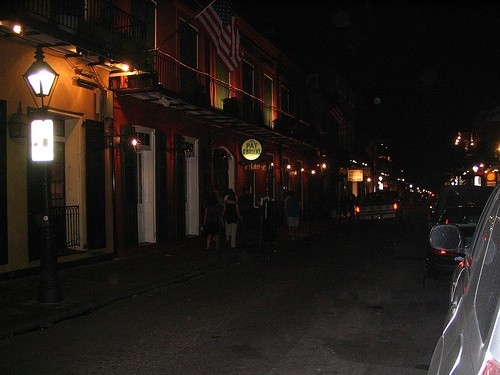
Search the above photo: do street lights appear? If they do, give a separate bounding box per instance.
[23,44,62,303]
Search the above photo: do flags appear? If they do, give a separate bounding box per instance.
[192,0,246,73]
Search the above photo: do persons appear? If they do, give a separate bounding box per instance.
[201,186,302,252]
[350,194,358,206]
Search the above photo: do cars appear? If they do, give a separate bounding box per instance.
[428,181,499,375]
[423,184,493,276]
[355,191,403,225]
